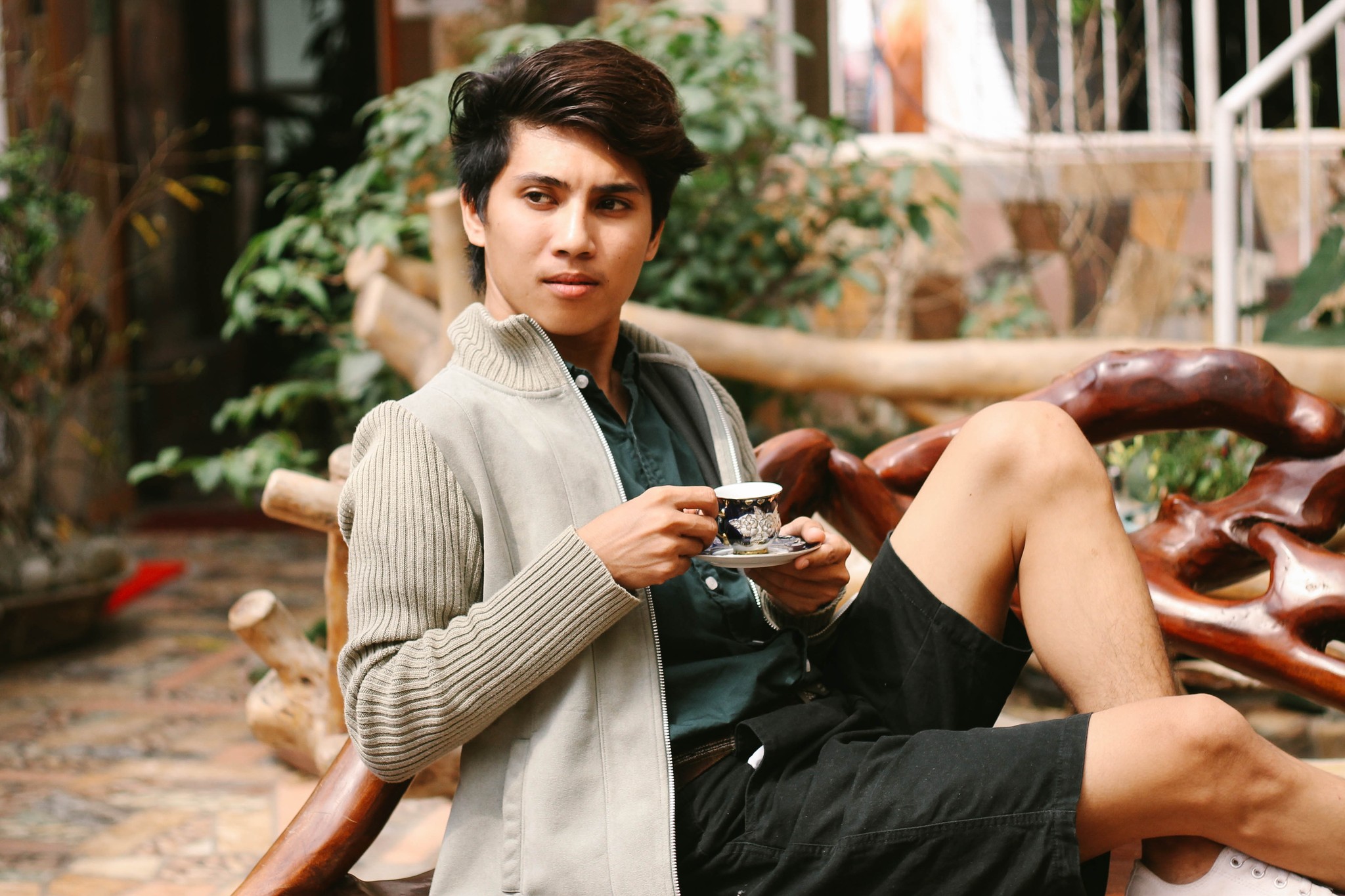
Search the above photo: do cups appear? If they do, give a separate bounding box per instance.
[694,481,783,554]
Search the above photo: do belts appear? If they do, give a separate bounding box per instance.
[673,681,825,790]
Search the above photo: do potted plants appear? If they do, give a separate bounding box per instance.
[2,97,134,658]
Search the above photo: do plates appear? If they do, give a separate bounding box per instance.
[693,535,822,568]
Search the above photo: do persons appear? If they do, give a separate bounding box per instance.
[336,38,1345,895]
